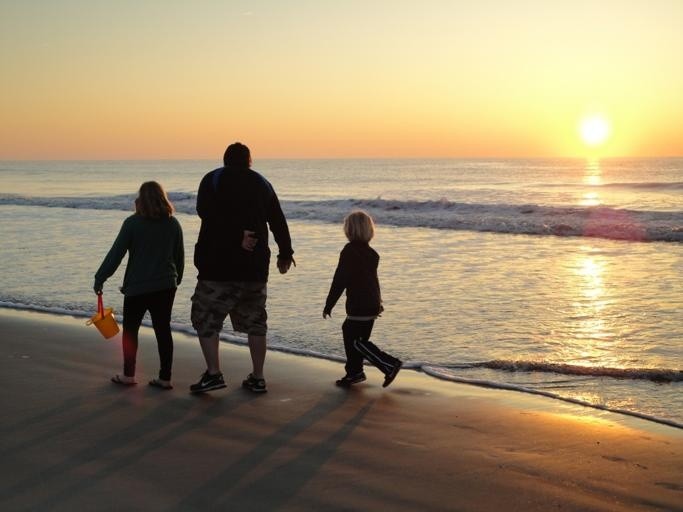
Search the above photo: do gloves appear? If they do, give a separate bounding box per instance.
[277,250,296,274]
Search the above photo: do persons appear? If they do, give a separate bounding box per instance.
[91,181,184,389]
[322,210,403,387]
[189,143,297,393]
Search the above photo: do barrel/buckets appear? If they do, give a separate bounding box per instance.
[85,293,120,339]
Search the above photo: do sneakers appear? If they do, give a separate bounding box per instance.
[383,358,403,388]
[336,371,366,387]
[242,372,268,392]
[190,369,228,394]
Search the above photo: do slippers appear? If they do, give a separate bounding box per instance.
[149,379,172,390]
[110,374,138,386]
